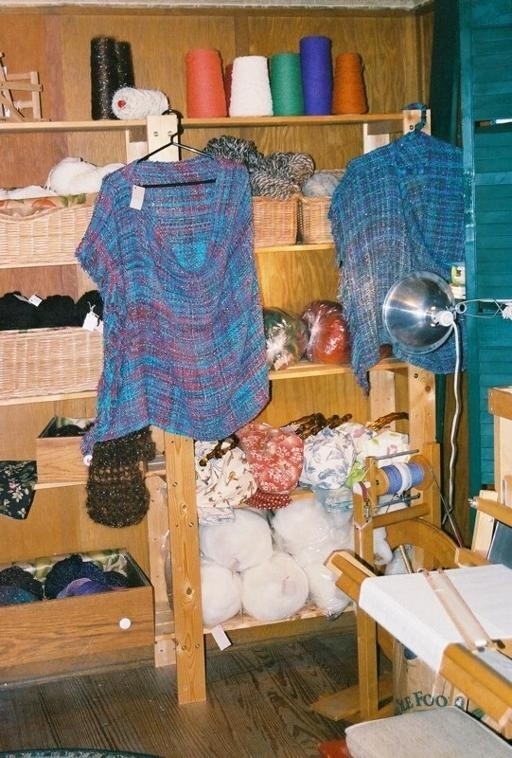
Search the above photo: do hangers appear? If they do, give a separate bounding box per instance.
[137,99,439,189]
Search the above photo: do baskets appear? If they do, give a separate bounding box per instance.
[1,320,111,404]
[299,195,341,247]
[0,190,100,267]
[246,192,299,250]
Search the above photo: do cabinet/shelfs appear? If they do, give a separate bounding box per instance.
[2,0,444,711]
[433,0,512,546]
